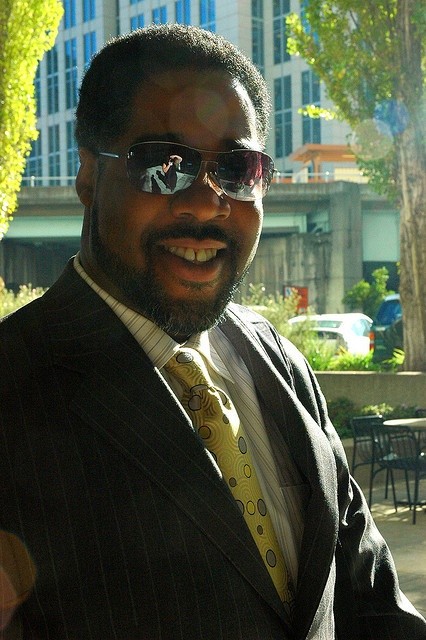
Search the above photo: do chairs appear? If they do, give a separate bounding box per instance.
[368,423,425,527]
[415,410,426,445]
[349,415,413,512]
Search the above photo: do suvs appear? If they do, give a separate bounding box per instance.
[370,294,403,367]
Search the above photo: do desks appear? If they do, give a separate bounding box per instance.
[383,417,426,511]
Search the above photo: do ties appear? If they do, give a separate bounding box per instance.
[166,346,291,614]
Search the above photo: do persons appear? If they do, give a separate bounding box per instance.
[1,24,425,633]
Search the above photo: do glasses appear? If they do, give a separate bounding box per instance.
[99,139,273,204]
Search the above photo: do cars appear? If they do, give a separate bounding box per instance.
[289,312,375,361]
